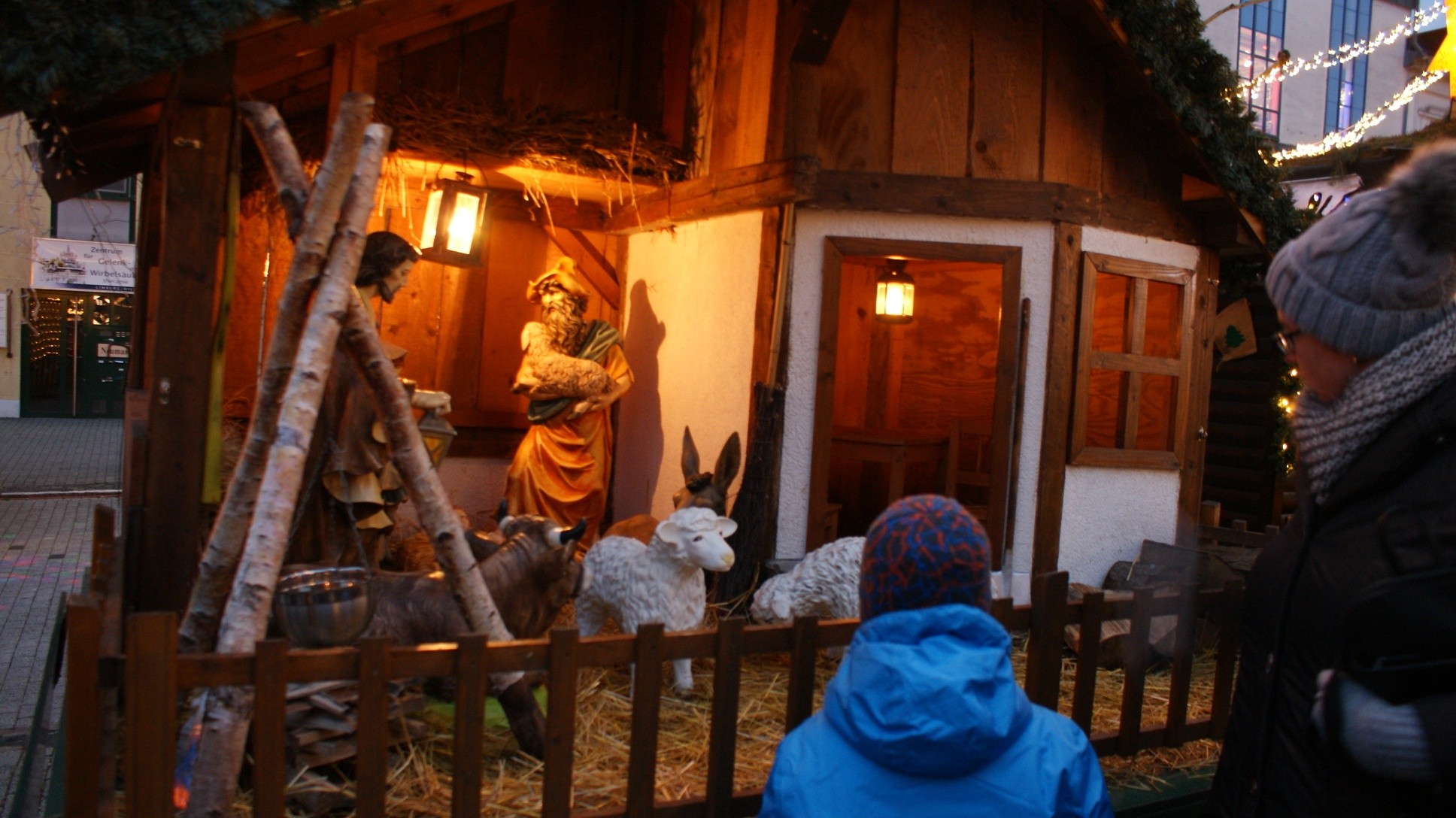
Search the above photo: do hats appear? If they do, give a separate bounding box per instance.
[1264,141,1455,355]
[859,494,991,622]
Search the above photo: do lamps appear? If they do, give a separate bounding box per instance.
[874,257,918,327]
[410,175,502,268]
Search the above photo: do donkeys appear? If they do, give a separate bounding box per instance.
[602,425,741,604]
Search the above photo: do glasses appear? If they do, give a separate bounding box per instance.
[1273,328,1301,353]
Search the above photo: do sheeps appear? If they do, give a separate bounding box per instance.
[749,536,872,668]
[573,507,739,700]
[509,320,616,421]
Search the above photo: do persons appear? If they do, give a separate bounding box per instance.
[502,256,635,551]
[1204,135,1456,817]
[290,231,450,567]
[756,493,1112,818]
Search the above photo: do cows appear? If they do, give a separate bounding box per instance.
[267,501,589,766]
